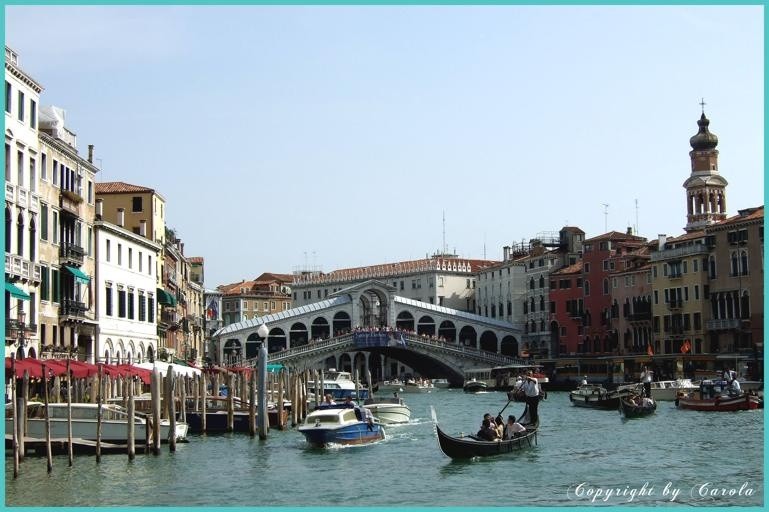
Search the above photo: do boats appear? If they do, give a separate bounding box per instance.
[306,367,369,400]
[297,401,386,445]
[463,363,549,391]
[383,378,451,394]
[568,383,646,409]
[363,394,411,425]
[616,377,701,401]
[617,393,657,417]
[463,381,488,393]
[5,399,190,443]
[694,378,762,395]
[673,389,763,412]
[507,387,548,402]
[435,406,540,457]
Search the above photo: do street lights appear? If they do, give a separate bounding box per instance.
[10,309,33,360]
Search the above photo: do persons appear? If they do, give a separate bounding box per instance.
[482,413,498,438]
[321,394,335,408]
[744,388,755,396]
[503,414,527,440]
[392,391,404,405]
[627,392,639,406]
[475,420,497,441]
[281,345,286,352]
[722,365,738,385]
[84,383,93,400]
[513,374,524,391]
[343,396,357,408]
[519,369,540,427]
[580,375,588,385]
[49,384,57,401]
[633,394,642,404]
[308,324,450,346]
[490,416,505,441]
[730,378,741,396]
[639,364,654,397]
[641,392,653,407]
[383,374,433,390]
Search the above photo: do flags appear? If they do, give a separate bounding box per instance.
[680,340,692,354]
[646,344,654,358]
